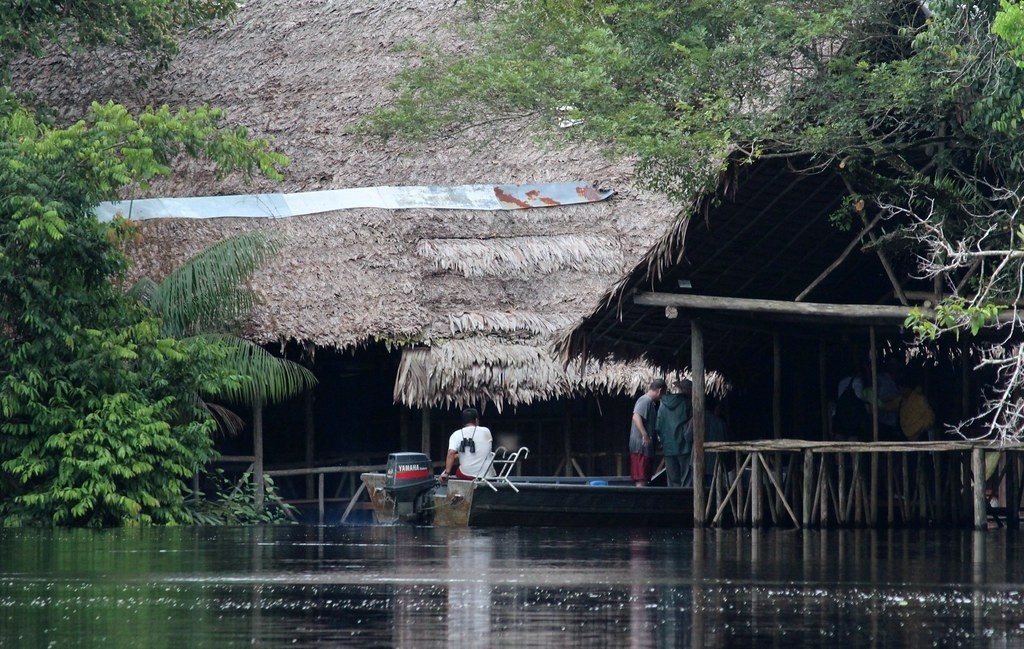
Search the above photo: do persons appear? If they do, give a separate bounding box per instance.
[438,408,498,484]
[685,367,944,488]
[655,379,693,487]
[628,378,668,487]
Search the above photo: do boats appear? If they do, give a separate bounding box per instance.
[360,470,786,528]
